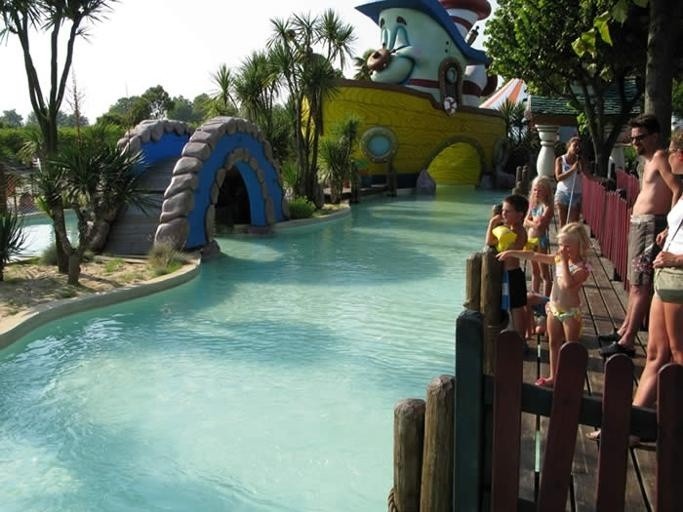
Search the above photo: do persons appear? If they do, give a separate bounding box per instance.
[585,122,683,449]
[594,112,683,359]
[484,136,590,355]
[494,221,591,389]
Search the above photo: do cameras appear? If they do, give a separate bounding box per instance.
[575,152,581,161]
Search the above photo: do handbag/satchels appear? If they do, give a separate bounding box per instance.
[655,266,683,304]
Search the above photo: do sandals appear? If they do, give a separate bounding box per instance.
[534,376,554,387]
[599,341,636,358]
[598,328,621,343]
